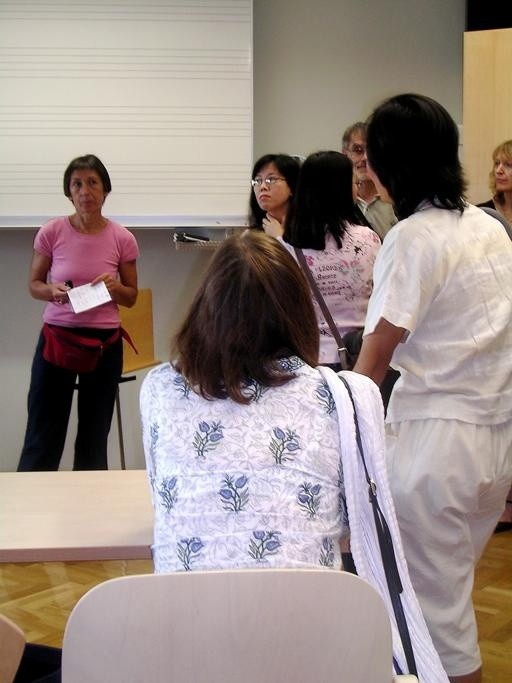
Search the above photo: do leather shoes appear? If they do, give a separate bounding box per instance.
[495,501,512,533]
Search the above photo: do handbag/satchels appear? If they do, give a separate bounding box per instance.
[342,329,400,419]
[42,328,106,374]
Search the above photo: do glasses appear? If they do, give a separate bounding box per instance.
[347,145,368,157]
[252,175,287,186]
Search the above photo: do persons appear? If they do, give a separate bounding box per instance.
[250,153,299,241]
[365,92,512,683]
[342,122,399,239]
[474,140,512,535]
[138,232,384,575]
[16,154,141,471]
[281,150,385,371]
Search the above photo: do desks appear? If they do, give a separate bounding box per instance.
[1,470,391,564]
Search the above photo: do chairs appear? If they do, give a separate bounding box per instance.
[1,611,29,683]
[61,570,419,683]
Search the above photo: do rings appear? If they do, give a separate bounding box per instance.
[58,299,62,305]
